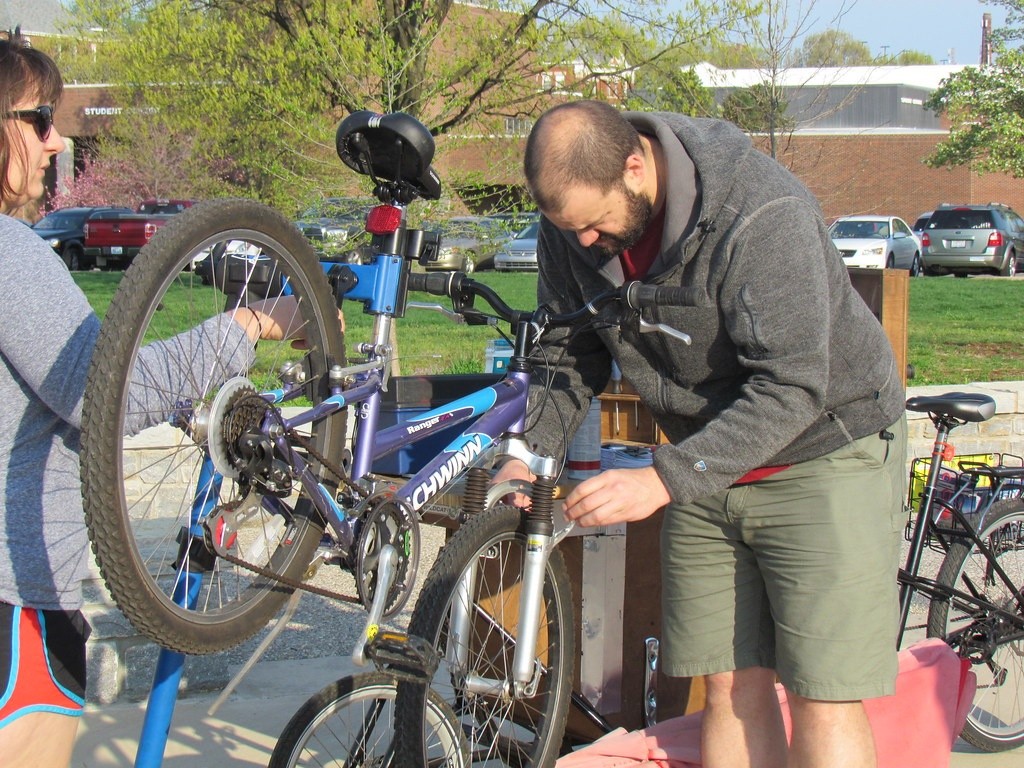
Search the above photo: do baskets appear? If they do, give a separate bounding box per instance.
[905,453,1024,554]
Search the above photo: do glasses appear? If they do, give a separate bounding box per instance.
[2,106,53,143]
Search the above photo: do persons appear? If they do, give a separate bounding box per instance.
[487,99,906,767]
[0,34,348,768]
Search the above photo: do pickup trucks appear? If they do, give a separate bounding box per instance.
[83,198,202,269]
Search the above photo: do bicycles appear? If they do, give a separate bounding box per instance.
[76,105,1023,768]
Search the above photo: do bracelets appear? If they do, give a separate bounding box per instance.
[240,305,262,339]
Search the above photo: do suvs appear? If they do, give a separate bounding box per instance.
[921,201,1024,278]
[28,205,133,271]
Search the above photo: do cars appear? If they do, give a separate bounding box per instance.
[293,197,384,268]
[494,219,541,271]
[422,215,516,273]
[824,215,924,278]
[483,212,537,232]
[912,211,934,240]
